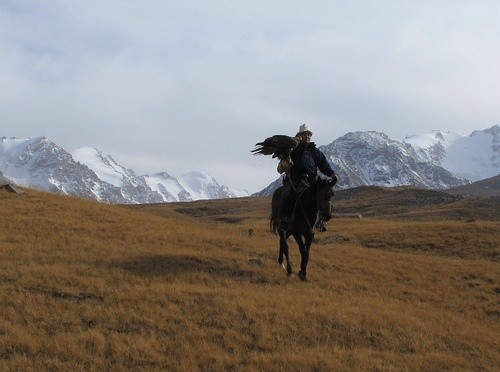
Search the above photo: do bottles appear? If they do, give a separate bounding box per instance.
[295,123,313,137]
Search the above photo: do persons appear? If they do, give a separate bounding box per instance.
[277,123,338,192]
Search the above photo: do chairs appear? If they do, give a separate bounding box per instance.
[318,222,328,232]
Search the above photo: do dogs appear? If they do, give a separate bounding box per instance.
[268,176,338,282]
